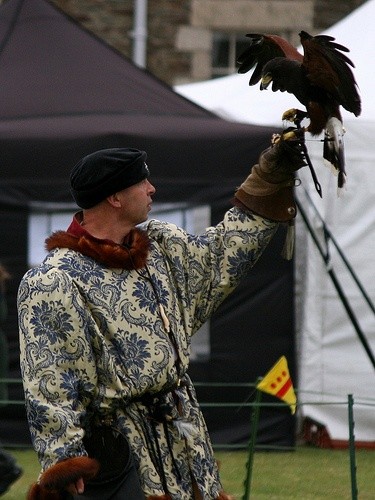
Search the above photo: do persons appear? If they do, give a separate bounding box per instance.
[16,127,304,500]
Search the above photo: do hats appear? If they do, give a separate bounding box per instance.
[69,148,149,209]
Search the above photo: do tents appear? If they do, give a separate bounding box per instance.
[169,1,374,448]
[0,0,299,448]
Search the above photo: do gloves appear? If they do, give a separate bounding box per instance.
[229,131,307,223]
